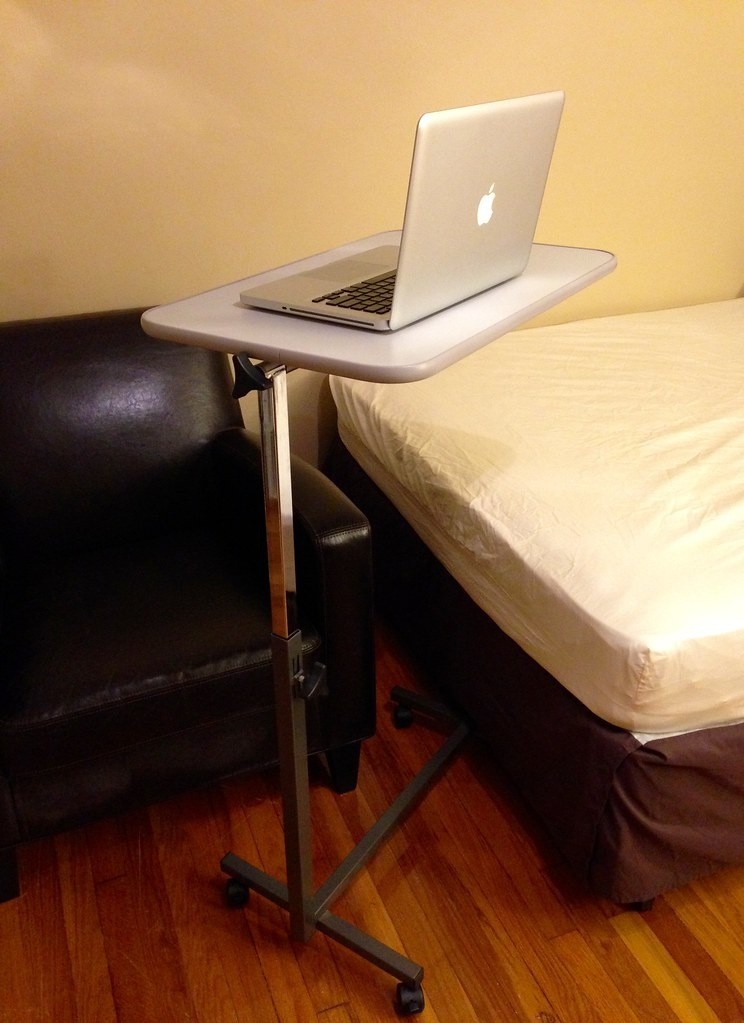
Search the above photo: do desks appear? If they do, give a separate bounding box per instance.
[140,230,616,1018]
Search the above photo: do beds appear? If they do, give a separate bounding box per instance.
[331,295,744,913]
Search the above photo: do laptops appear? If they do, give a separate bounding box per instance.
[240,89,565,333]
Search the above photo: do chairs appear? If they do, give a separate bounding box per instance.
[0,307,373,905]
